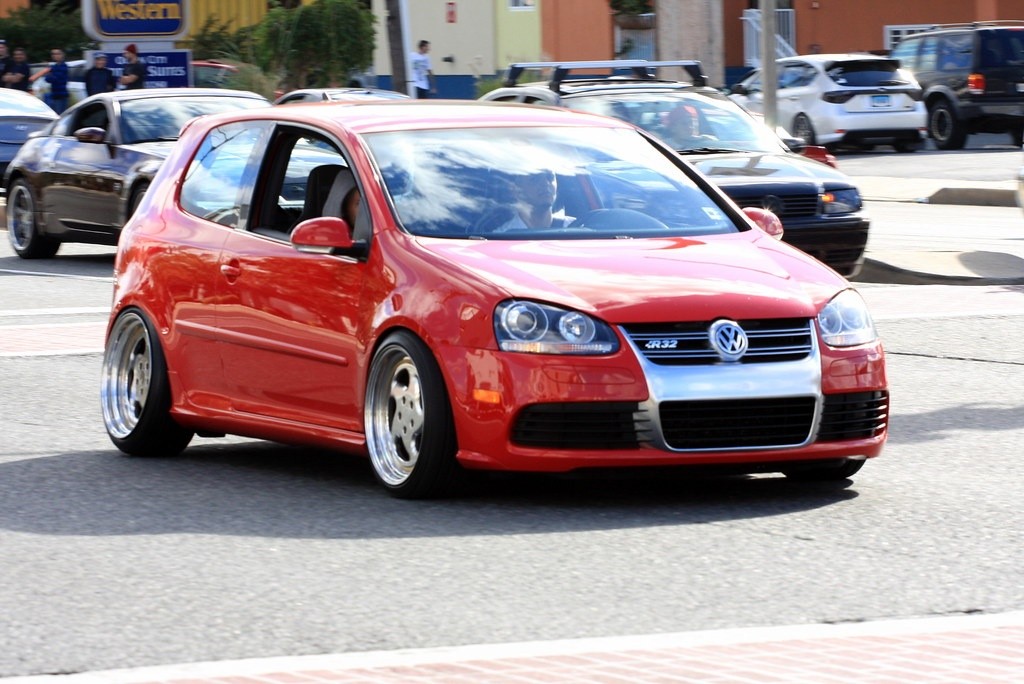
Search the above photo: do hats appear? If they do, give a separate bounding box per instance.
[126,44,136,54]
[92,51,107,58]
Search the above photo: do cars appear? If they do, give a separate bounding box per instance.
[30,63,88,100]
[0,85,60,196]
[98,98,889,499]
[189,61,265,90]
[277,87,416,100]
[5,88,415,259]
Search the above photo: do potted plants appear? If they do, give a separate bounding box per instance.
[609,0,657,31]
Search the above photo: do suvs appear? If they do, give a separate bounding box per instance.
[893,17,1024,151]
[728,52,928,151]
[470,61,870,279]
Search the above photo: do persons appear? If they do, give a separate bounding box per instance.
[329,187,372,263]
[29,47,71,115]
[409,39,437,99]
[120,42,147,88]
[0,39,30,92]
[472,168,584,237]
[84,51,116,97]
[663,106,720,149]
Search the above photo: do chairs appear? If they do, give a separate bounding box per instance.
[465,163,531,235]
[303,163,353,231]
